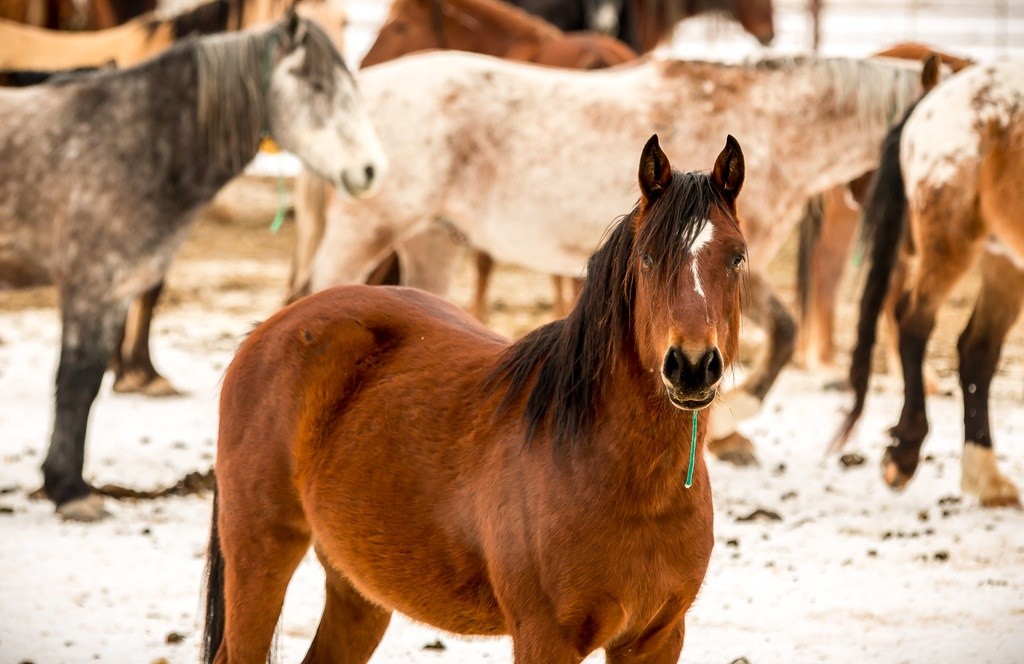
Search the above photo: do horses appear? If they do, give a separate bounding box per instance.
[0,0,1024,520]
[196,134,753,664]
